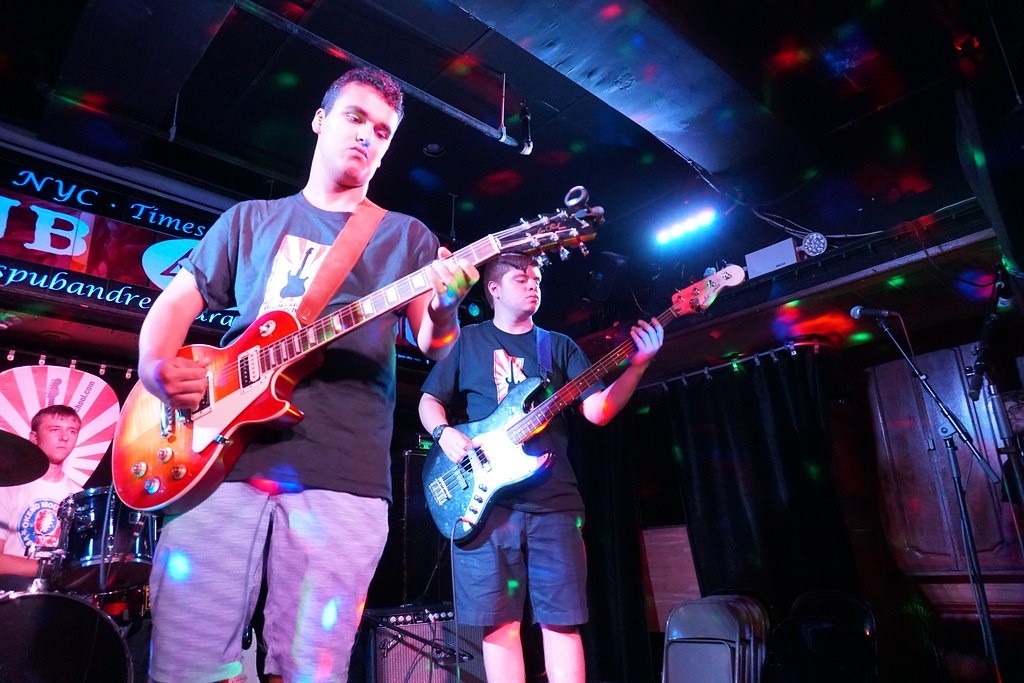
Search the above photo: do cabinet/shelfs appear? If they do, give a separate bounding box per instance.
[864,340,1024,619]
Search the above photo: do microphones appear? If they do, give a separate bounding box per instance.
[439,652,473,664]
[850,305,899,318]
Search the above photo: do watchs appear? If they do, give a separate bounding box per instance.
[432,424,448,441]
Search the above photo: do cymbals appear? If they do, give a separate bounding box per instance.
[0,429,51,487]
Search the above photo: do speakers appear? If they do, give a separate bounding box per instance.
[375,447,454,603]
[360,600,488,683]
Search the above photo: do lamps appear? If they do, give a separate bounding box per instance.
[744,232,827,279]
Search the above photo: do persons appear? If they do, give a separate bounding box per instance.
[139,69,481,683]
[417,251,664,683]
[1000,391,1024,544]
[0,404,98,609]
[830,363,906,603]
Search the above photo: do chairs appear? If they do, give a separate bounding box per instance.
[664,594,768,683]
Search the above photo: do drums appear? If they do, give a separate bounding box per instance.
[0,585,136,683]
[51,484,161,598]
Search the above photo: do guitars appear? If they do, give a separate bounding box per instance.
[108,184,607,520]
[419,257,749,549]
